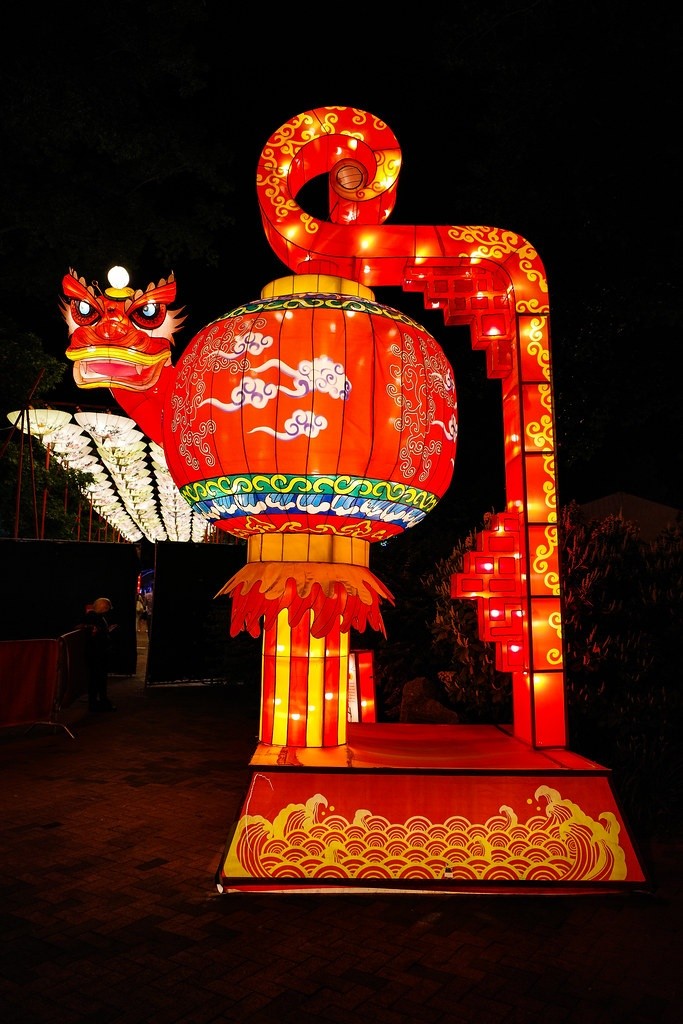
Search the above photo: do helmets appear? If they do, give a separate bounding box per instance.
[94,597,113,614]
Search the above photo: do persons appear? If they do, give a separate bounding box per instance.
[76,598,116,719]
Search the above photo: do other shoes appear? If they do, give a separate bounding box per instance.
[90,702,104,712]
[100,700,117,711]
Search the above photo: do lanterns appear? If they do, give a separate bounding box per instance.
[163,275,456,752]
